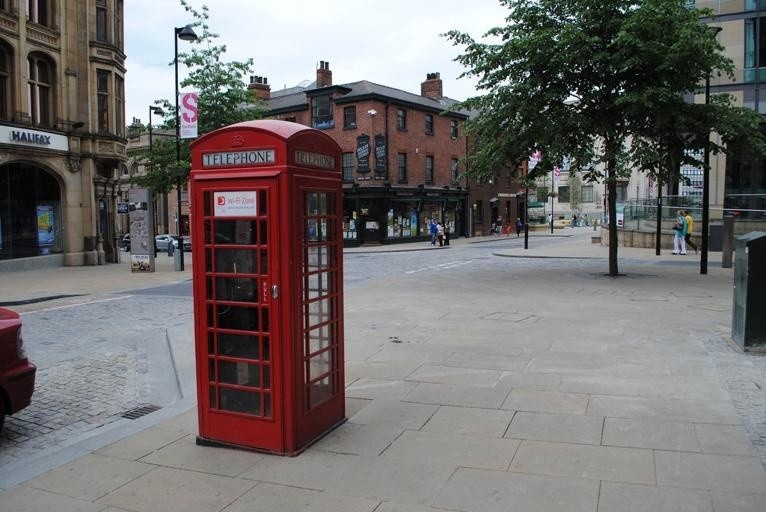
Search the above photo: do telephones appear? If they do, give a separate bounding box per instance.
[217,242,257,307]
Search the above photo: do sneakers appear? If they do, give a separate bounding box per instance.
[671,247,697,255]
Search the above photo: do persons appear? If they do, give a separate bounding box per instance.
[429,218,437,246]
[443,223,449,246]
[496,215,504,237]
[670,209,687,256]
[514,217,523,237]
[546,214,552,226]
[435,222,444,247]
[680,210,699,255]
[571,213,576,228]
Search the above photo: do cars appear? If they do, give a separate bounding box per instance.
[1,308,36,434]
[154,234,191,252]
[124,233,131,252]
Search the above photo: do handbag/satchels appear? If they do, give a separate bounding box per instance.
[671,217,683,231]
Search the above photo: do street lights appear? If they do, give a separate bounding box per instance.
[175,27,197,270]
[700,27,723,273]
[150,106,163,177]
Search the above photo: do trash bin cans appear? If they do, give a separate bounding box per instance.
[709,224,723,251]
[618,220,622,225]
